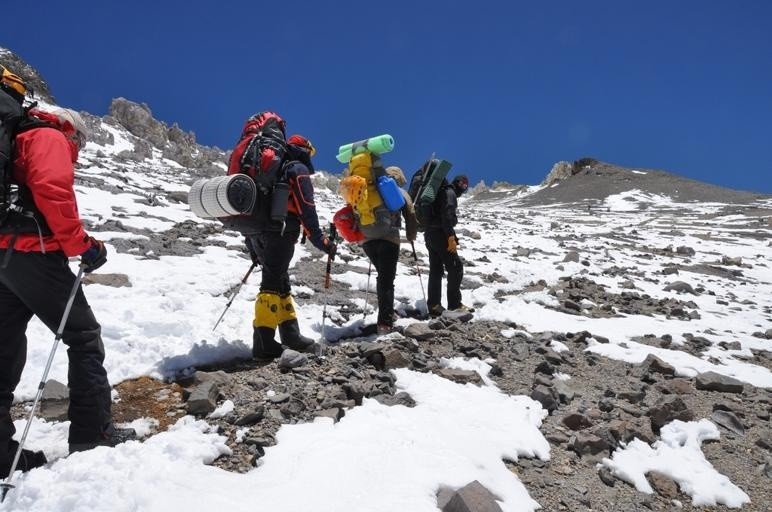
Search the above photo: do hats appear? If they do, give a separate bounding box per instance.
[385,167,407,188]
[452,175,468,196]
[287,136,315,157]
[51,108,85,150]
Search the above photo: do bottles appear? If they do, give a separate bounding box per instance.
[375,170,406,212]
[271,180,289,220]
[9,184,19,206]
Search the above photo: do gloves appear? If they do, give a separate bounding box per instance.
[319,236,336,261]
[405,216,419,239]
[78,236,107,272]
[447,236,456,253]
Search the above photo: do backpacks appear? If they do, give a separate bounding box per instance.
[1,65,60,268]
[339,153,400,239]
[218,112,302,236]
[408,159,447,232]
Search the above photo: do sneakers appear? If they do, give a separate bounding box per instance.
[448,304,474,312]
[1,450,47,480]
[377,325,390,335]
[428,304,445,317]
[391,312,400,322]
[69,421,136,453]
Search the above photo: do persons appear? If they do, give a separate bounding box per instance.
[359,165,415,335]
[249,133,337,358]
[0,104,136,479]
[426,176,476,320]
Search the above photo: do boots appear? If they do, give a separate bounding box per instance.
[278,292,314,352]
[252,290,289,361]
